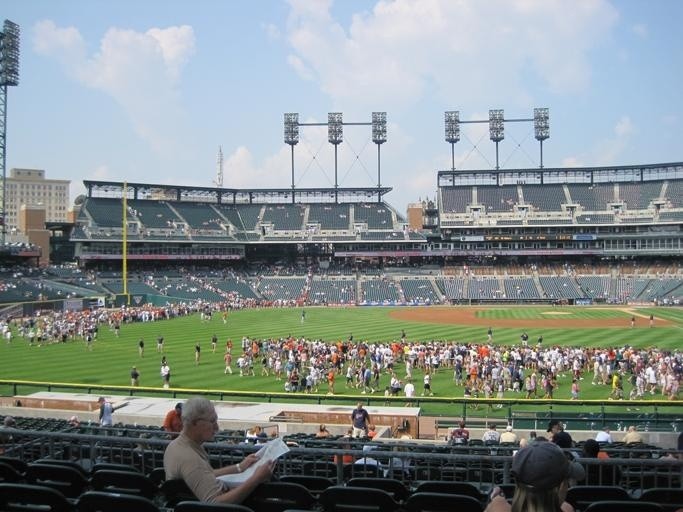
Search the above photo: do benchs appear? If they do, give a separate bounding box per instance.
[434,419,507,440]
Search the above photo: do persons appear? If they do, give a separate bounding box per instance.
[0,196,683,512]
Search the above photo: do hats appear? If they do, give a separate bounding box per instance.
[510,442,585,487]
